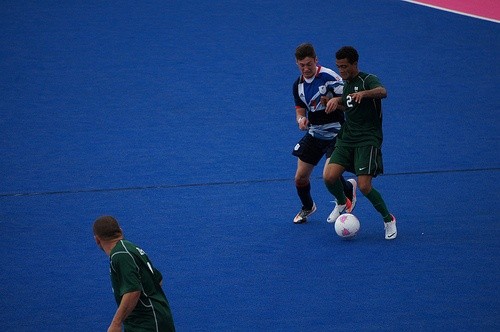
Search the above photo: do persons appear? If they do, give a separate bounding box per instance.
[93,216,176,332]
[321,46,398,240]
[291,43,358,223]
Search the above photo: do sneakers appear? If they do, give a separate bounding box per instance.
[346,178,357,212]
[327,198,352,223]
[293,202,317,223]
[384,213,397,239]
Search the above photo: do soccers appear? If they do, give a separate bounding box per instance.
[335,214,360,238]
